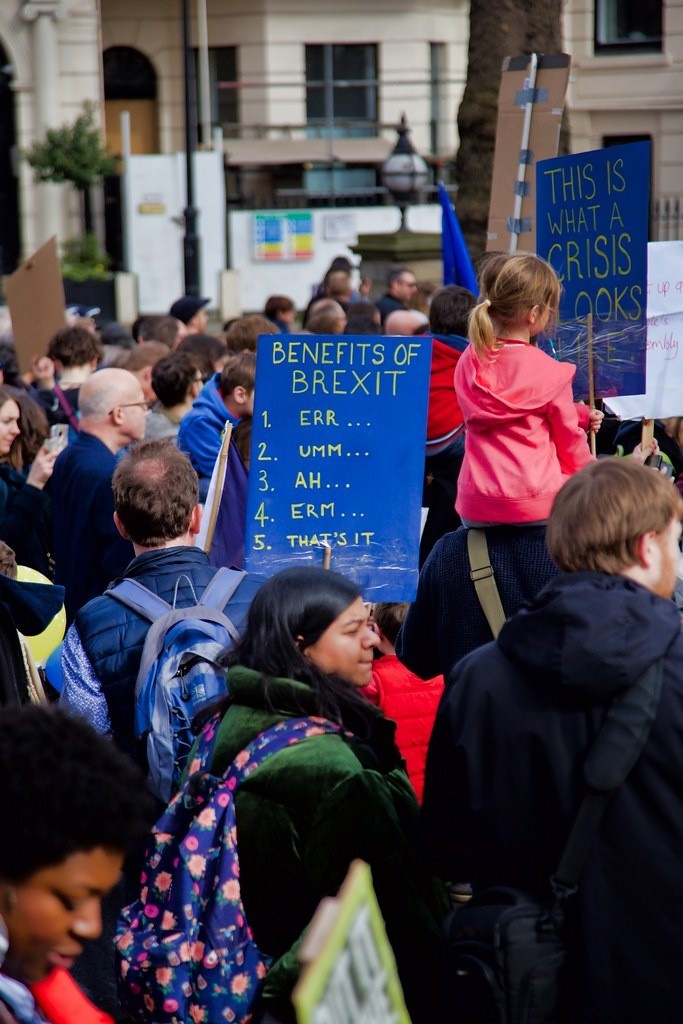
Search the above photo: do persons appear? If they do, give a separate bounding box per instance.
[0,252,683,802]
[174,567,421,1024]
[0,692,160,1024]
[421,457,683,1024]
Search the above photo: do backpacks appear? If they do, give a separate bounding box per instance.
[112,701,359,1024]
[103,565,248,804]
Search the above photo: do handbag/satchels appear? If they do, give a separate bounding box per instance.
[442,886,566,1024]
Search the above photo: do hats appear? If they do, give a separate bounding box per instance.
[170,297,211,324]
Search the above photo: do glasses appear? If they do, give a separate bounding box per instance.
[192,373,209,382]
[108,399,150,415]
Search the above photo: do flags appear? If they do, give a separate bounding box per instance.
[439,181,481,300]
